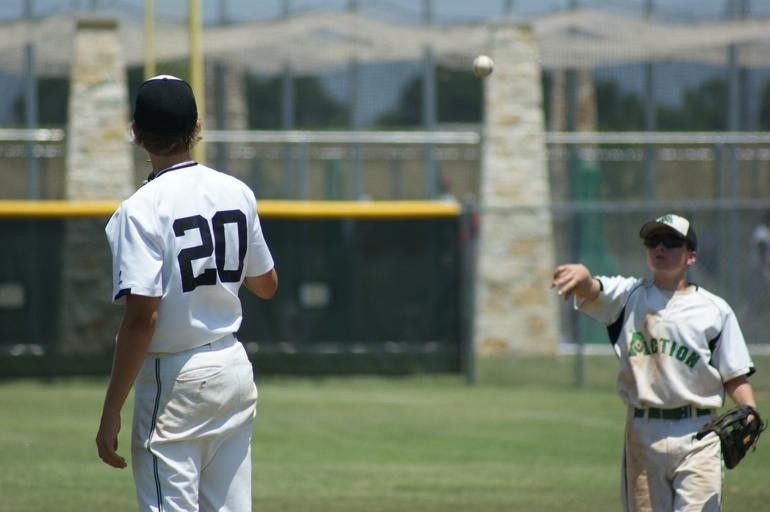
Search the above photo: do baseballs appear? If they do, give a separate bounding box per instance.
[472,56,494,77]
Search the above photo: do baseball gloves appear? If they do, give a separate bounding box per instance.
[692,405,768,469]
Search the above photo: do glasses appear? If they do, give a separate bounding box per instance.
[643,233,686,247]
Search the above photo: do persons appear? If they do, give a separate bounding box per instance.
[95,74,278,512]
[549,213,762,512]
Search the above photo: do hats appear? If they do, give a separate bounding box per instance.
[640,213,697,247]
[134,75,198,128]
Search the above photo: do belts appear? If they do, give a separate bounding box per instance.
[206,332,239,346]
[634,405,712,419]
